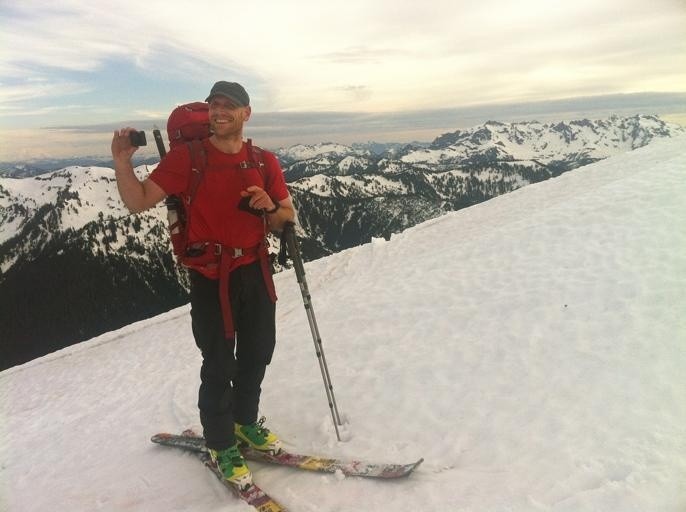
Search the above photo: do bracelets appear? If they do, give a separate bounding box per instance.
[264,199,280,214]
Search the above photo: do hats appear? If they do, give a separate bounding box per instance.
[205,81,249,105]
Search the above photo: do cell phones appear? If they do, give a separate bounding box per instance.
[129,130,147,146]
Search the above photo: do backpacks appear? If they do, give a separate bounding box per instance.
[168,102,270,264]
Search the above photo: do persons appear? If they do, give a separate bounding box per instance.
[111,81,295,486]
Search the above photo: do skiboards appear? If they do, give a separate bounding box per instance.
[152,428,424,512]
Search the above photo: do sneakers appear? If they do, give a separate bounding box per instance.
[207,442,253,492]
[233,416,282,456]
